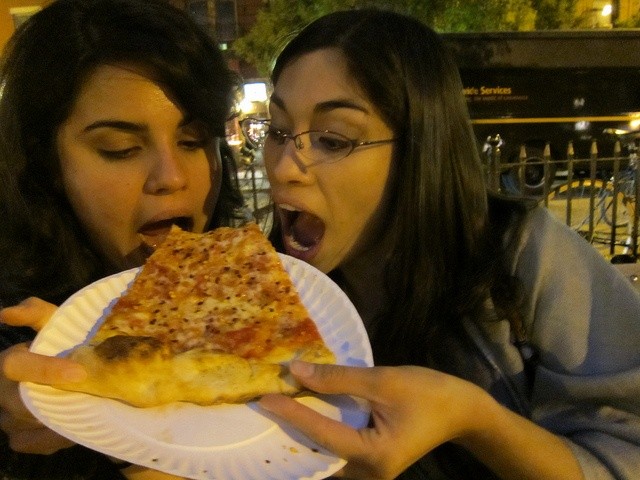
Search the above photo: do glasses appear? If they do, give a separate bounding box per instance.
[238,117,397,164]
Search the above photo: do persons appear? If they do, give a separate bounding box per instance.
[2,8,639,479]
[2,1,246,479]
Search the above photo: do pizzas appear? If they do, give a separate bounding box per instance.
[48,220,337,410]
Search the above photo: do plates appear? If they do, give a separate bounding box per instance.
[17,251,375,479]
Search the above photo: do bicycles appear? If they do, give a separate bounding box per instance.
[536,128,640,264]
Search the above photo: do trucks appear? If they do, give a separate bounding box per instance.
[435,29,640,198]
